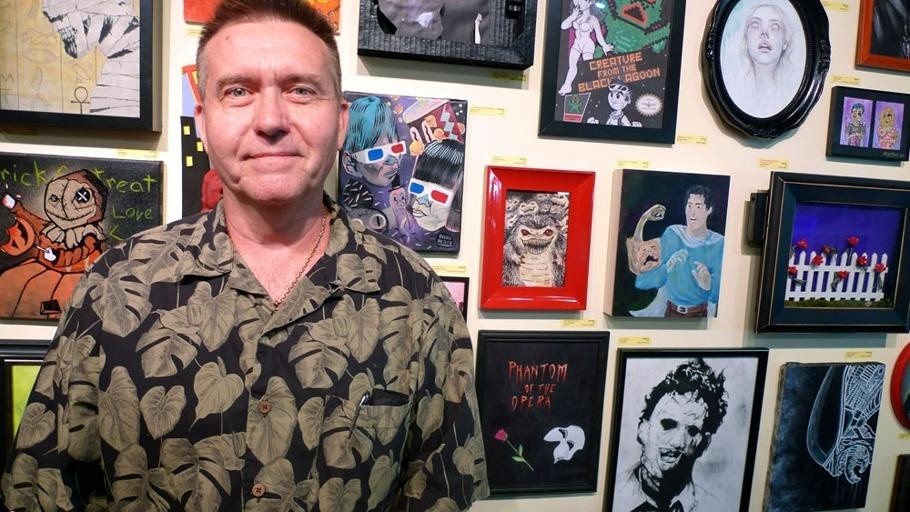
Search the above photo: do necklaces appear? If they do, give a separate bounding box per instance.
[273,201,330,310]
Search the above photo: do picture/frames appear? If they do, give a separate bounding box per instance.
[889,342,910,436]
[356,0,539,70]
[753,168,910,336]
[598,346,772,512]
[699,0,834,147]
[475,328,609,497]
[437,275,470,326]
[0,339,58,476]
[854,1,910,73]
[825,86,910,163]
[537,0,687,145]
[477,165,595,312]
[0,1,166,135]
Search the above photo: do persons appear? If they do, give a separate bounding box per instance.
[0,0,493,512]
[735,2,802,102]
[630,185,727,317]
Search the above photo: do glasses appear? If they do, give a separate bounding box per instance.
[352,140,406,165]
[406,178,456,208]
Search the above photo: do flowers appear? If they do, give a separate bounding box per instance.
[490,426,539,475]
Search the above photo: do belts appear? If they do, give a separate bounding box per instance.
[667,302,707,314]
